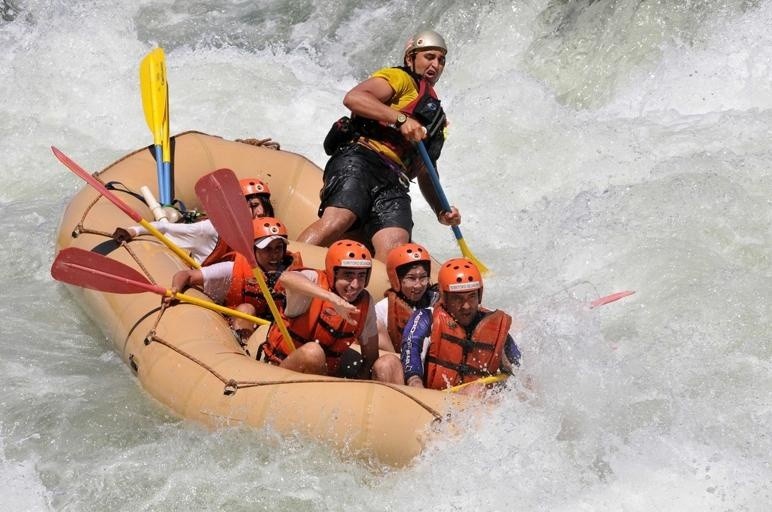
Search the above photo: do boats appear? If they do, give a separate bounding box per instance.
[56,128,509,471]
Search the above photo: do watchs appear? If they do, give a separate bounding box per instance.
[394,112,408,129]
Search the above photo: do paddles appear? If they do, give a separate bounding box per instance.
[140,48,164,209]
[195,169,296,352]
[161,82,172,208]
[409,126,490,278]
[590,291,634,308]
[50,145,203,271]
[51,248,274,326]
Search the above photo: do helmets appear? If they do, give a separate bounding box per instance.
[253,217,289,240]
[438,259,483,306]
[387,243,432,292]
[404,30,447,64]
[326,239,372,291]
[239,178,271,197]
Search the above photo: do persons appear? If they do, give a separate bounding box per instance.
[372,260,538,398]
[295,29,461,262]
[255,241,379,380]
[114,177,275,271]
[375,241,439,357]
[160,218,305,356]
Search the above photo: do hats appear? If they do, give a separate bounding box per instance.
[254,236,290,250]
